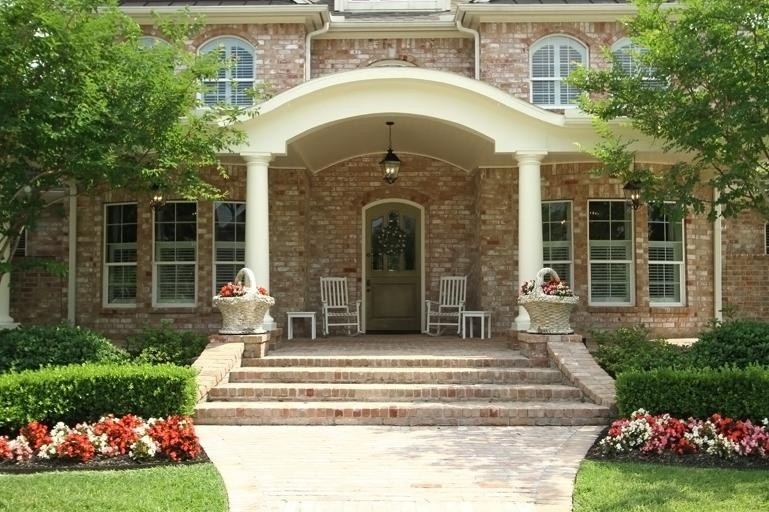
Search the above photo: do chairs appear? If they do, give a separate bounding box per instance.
[319,276,363,336]
[424,274,467,336]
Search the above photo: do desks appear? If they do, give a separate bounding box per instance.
[286,311,317,340]
[462,310,492,340]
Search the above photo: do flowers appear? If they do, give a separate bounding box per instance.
[219,281,266,297]
[522,279,573,298]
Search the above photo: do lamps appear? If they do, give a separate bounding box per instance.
[378,121,401,185]
